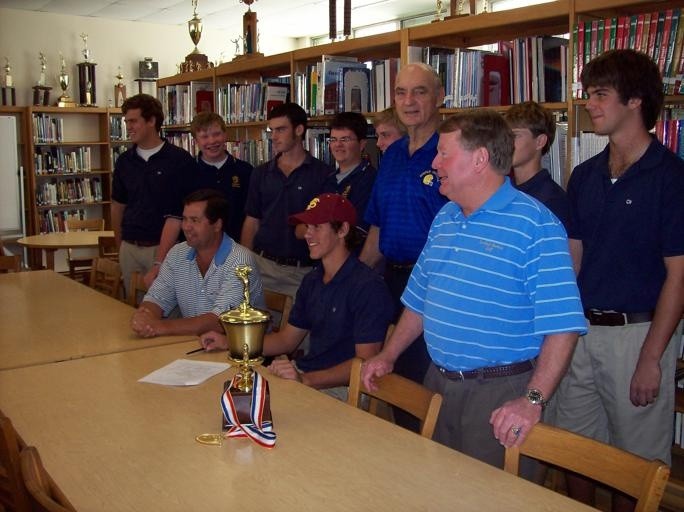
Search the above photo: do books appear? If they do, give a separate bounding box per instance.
[30,111,92,175]
[214,55,400,166]
[109,115,130,173]
[157,82,216,158]
[36,176,103,231]
[406,12,682,192]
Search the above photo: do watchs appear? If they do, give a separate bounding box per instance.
[523,388,547,411]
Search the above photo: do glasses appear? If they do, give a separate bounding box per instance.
[328,136,351,143]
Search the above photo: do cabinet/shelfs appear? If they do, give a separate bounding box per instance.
[19,101,134,272]
[153,0,684,196]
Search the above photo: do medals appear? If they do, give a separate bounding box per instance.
[195,433,226,448]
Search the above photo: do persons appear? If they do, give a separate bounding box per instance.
[360,109,592,485]
[202,195,397,389]
[108,62,582,307]
[567,48,683,511]
[129,190,268,340]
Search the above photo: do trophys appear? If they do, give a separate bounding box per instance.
[430,1,490,24]
[178,1,214,73]
[233,0,264,62]
[1,32,128,107]
[220,262,274,434]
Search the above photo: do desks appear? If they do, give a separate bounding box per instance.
[0,267,202,380]
[0,337,610,512]
[16,226,118,272]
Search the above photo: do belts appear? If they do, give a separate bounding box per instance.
[586,310,654,326]
[435,360,531,382]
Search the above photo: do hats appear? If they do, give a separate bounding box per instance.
[289,193,357,224]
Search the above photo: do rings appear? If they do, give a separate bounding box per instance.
[511,425,520,435]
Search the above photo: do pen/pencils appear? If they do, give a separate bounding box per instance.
[187,348,205,354]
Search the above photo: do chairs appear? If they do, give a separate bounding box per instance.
[0,253,23,275]
[364,322,395,416]
[344,357,445,439]
[0,414,32,512]
[18,440,77,512]
[502,417,673,512]
[95,235,121,264]
[125,270,149,309]
[258,288,294,332]
[87,255,122,300]
[57,217,105,280]
